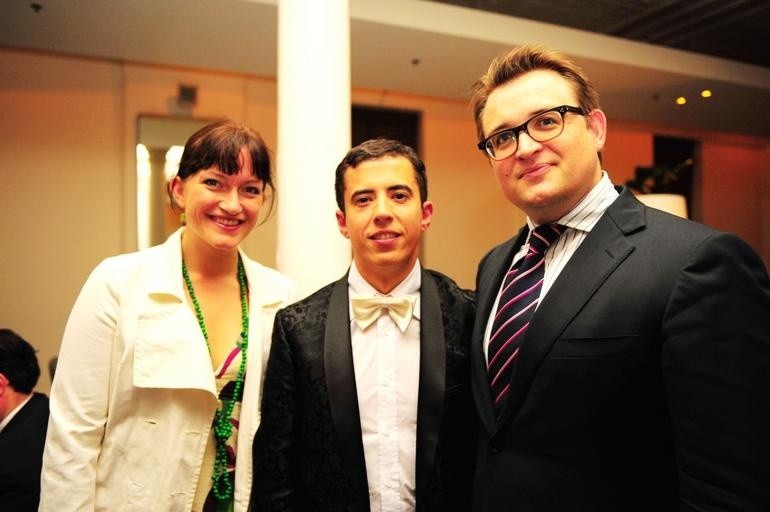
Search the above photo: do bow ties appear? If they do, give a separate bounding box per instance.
[351,295,418,333]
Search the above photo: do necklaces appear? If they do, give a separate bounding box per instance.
[182,254,249,502]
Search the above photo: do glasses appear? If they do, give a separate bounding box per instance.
[476,105,584,161]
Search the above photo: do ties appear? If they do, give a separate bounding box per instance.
[487,223,568,415]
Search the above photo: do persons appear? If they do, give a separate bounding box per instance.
[0,327,49,512]
[248,138,477,512]
[465,44,768,511]
[38,120,320,512]
